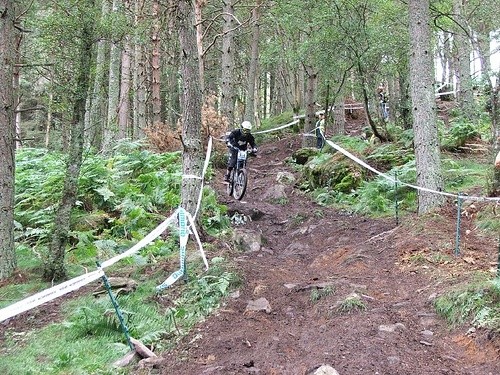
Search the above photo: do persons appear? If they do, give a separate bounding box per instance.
[377,86,388,119]
[224,120,257,182]
[314,112,326,149]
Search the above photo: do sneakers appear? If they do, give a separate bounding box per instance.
[223,174,230,184]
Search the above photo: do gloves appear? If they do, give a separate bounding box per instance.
[227,142,233,148]
[252,148,257,153]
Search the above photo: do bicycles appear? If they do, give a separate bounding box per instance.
[224,144,257,201]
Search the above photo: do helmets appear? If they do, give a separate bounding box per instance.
[240,121,253,136]
